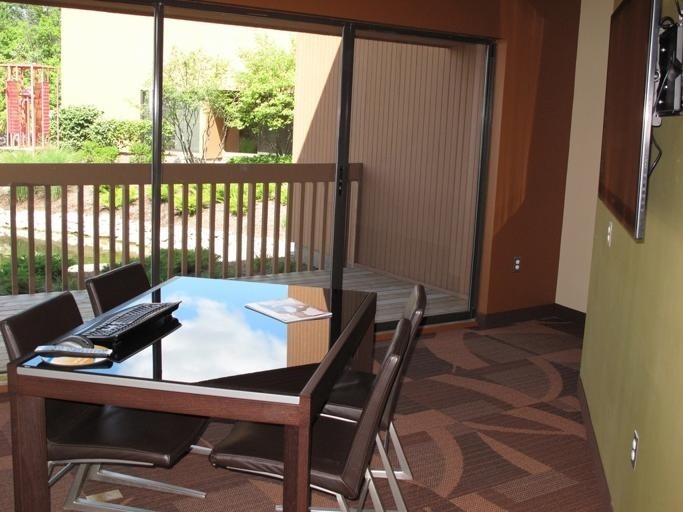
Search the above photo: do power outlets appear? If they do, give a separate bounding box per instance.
[512,256,520,273]
[630,430,639,470]
[607,222,612,247]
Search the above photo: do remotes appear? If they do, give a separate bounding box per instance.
[34,345,113,358]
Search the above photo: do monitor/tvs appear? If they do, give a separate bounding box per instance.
[597,0,662,243]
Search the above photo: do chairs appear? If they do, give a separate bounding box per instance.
[320,285,426,482]
[0,290,207,510]
[207,318,409,511]
[84,262,220,499]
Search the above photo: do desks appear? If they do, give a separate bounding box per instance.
[5,276,377,511]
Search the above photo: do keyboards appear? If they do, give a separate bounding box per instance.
[80,300,183,343]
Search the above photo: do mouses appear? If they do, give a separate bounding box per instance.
[56,334,94,349]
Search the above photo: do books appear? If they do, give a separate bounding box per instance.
[243,296,333,324]
